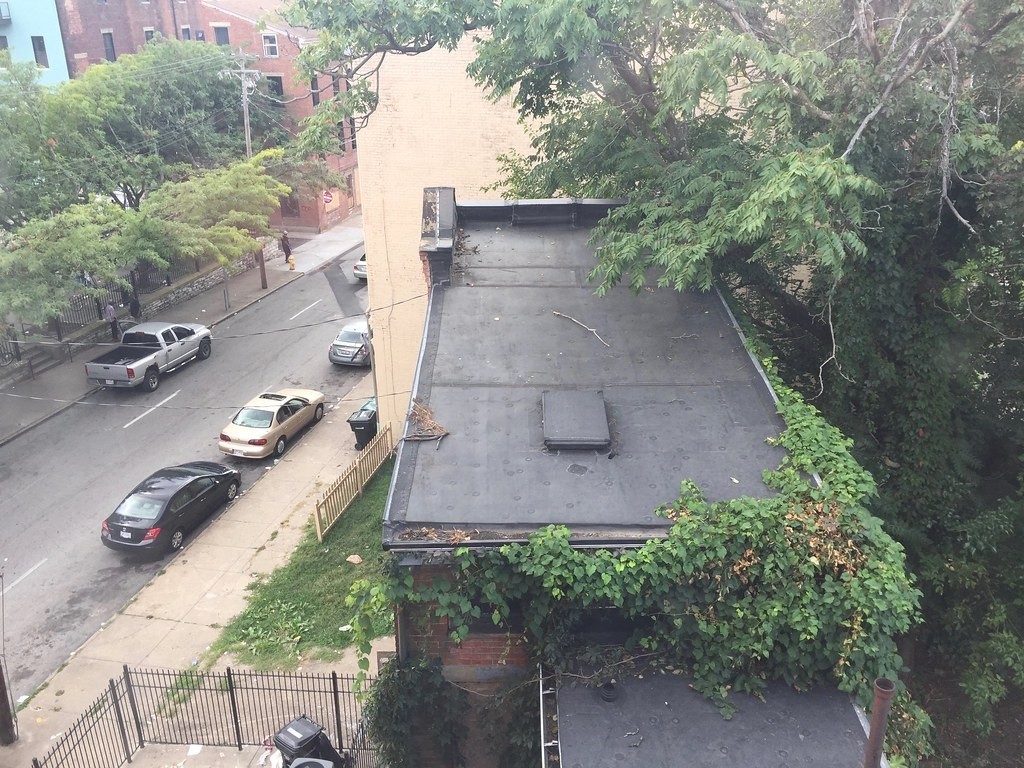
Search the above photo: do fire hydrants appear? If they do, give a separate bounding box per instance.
[288,255,296,270]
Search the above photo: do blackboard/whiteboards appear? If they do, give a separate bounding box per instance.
[377,650,396,673]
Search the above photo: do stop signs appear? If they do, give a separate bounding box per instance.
[323,190,334,205]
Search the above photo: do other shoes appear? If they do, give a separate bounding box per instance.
[286,261,290,263]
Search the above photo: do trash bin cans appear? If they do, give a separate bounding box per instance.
[347,410,377,451]
[272,711,326,768]
[289,757,334,768]
[358,395,376,410]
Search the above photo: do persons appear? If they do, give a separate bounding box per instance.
[281,231,291,263]
[106,299,120,341]
[131,295,142,324]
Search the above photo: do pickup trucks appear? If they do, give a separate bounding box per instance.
[84,321,215,393]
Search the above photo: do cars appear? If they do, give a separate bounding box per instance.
[353,253,367,279]
[328,319,373,365]
[101,460,242,555]
[217,388,326,459]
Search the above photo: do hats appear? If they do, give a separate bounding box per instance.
[283,231,288,235]
